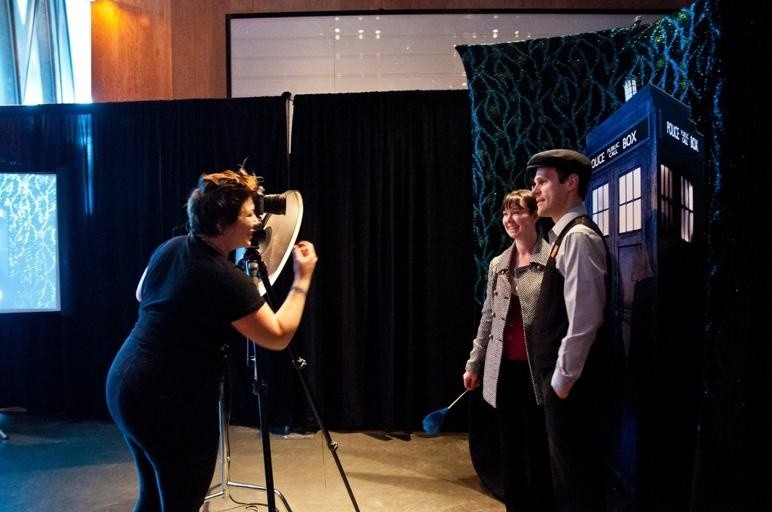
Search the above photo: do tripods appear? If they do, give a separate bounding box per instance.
[202,246,360,512]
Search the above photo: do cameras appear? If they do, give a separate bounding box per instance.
[249,187,286,217]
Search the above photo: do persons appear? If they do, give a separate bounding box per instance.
[522,148,626,512]
[105,170,319,512]
[289,286,308,297]
[463,189,553,512]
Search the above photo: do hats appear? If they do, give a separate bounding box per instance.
[526,148,593,176]
